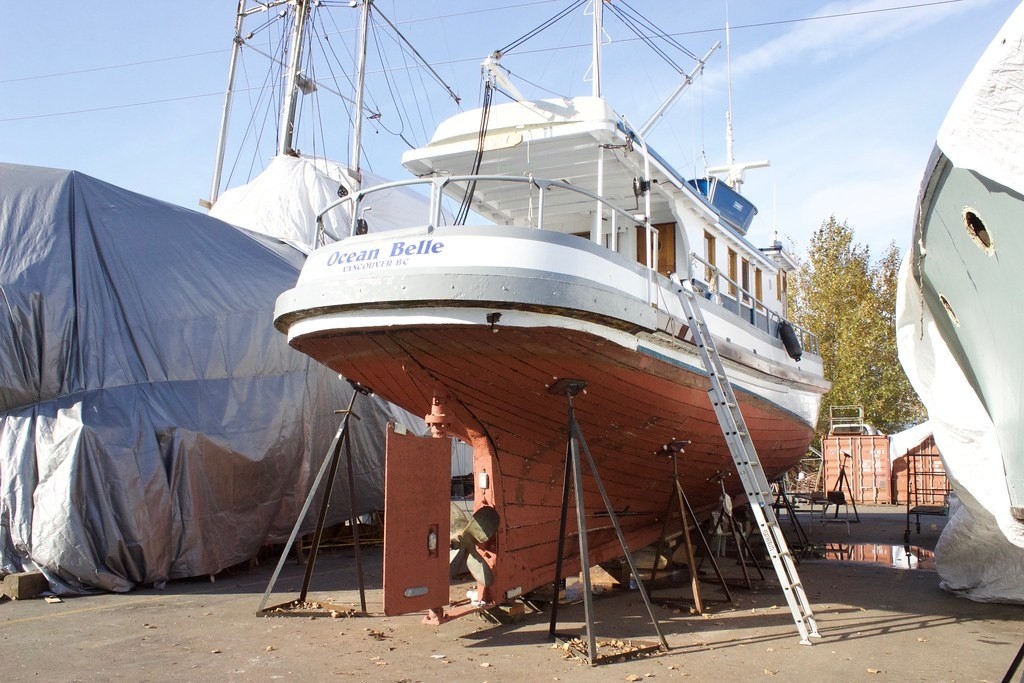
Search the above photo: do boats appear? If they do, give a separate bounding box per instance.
[894,1,1024,535]
[269,0,830,604]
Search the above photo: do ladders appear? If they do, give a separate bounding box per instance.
[665,273,823,648]
